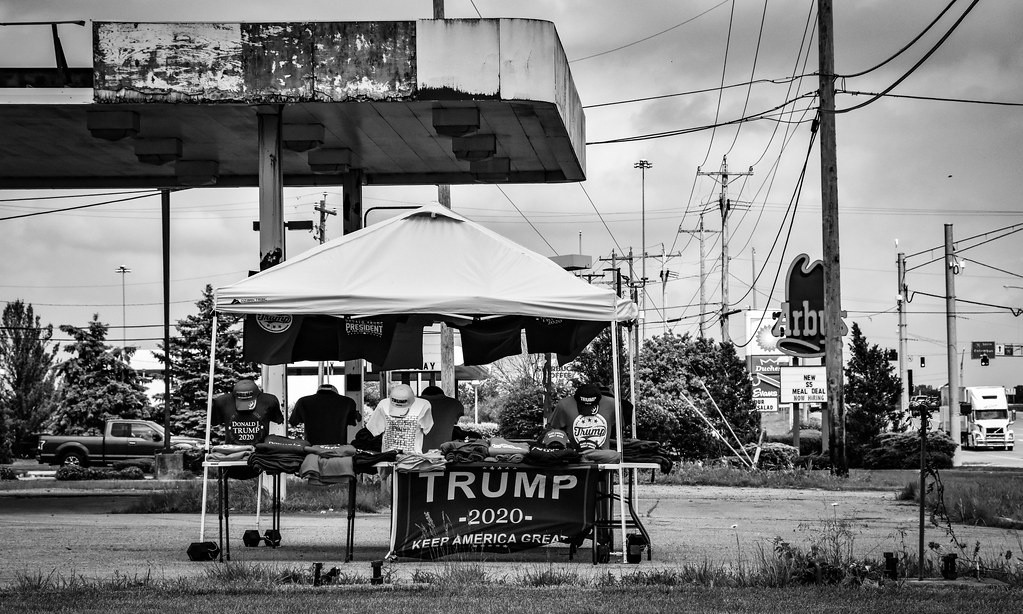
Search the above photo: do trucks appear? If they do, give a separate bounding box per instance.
[939,385,1017,451]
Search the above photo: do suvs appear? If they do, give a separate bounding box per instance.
[911,395,940,414]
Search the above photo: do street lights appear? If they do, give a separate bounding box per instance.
[113,265,132,366]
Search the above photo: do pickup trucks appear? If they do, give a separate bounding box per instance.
[35,419,221,476]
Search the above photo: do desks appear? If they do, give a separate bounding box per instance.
[391,461,659,565]
[201,460,389,562]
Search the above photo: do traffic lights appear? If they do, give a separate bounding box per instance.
[981,356,990,366]
[920,357,926,368]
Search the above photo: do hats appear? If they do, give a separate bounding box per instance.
[574,384,602,416]
[543,429,567,446]
[233,380,258,410]
[389,384,415,418]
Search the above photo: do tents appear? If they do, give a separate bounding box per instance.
[199,200,639,564]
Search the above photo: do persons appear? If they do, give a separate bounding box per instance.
[549,384,633,450]
[365,385,434,454]
[417,386,464,453]
[212,379,284,446]
[289,384,356,445]
